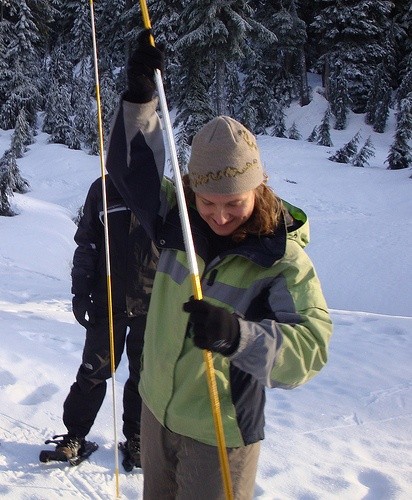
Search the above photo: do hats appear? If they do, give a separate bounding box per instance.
[187,116,262,194]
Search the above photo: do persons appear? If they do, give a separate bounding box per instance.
[39,172,161,472]
[105,27,333,500]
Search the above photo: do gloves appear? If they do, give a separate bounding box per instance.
[122,29,162,104]
[72,295,99,325]
[182,294,240,356]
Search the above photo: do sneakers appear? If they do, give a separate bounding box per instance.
[123,433,141,468]
[44,432,85,461]
